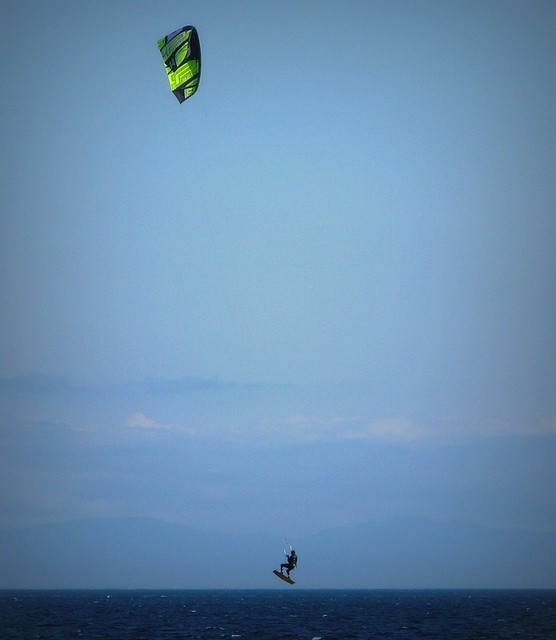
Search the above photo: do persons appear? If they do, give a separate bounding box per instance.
[279,550,298,579]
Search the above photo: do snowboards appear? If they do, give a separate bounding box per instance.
[273,570,295,584]
